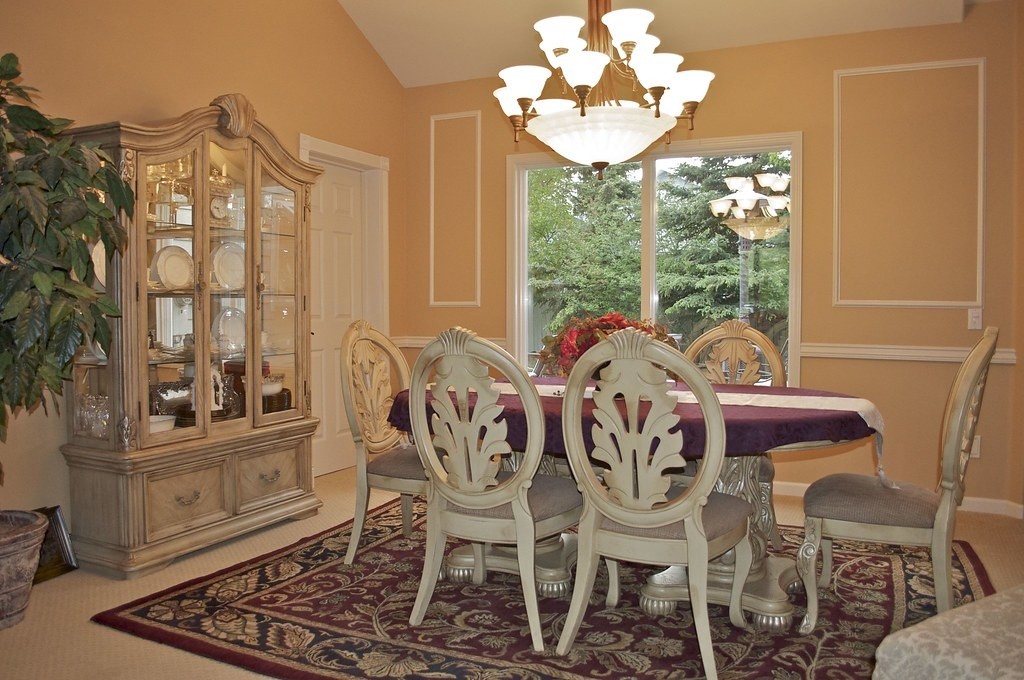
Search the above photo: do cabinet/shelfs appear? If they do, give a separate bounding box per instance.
[55,93,321,582]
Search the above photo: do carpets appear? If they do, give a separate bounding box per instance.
[92,492,998,680]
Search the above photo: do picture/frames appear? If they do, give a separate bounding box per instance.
[29,504,80,585]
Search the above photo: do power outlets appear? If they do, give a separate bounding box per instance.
[970,436,982,460]
[968,310,983,330]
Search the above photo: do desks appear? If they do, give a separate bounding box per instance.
[384,376,885,629]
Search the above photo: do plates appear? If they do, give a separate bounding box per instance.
[211,308,224,340]
[219,309,245,350]
[213,242,246,290]
[209,242,224,284]
[150,247,163,283]
[156,246,196,288]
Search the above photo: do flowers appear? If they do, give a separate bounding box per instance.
[542,308,684,383]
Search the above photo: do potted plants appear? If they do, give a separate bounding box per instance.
[0,51,135,630]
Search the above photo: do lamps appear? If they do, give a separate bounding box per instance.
[706,166,791,242]
[494,0,715,181]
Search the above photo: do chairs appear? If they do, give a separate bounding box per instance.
[555,326,756,680]
[797,327,1001,635]
[674,319,786,550]
[401,324,585,652]
[340,319,448,566]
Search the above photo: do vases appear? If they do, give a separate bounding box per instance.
[591,361,625,401]
